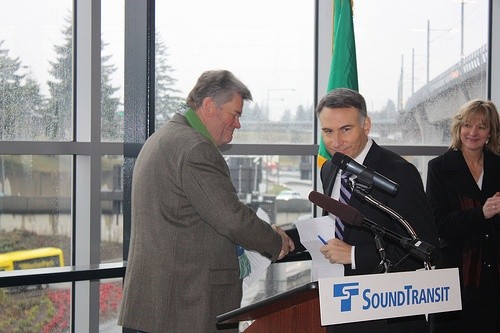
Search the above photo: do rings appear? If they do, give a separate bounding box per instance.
[322,245,326,252]
[493,203,497,209]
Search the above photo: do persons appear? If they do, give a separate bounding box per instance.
[117,69,295,333]
[284,87,441,332]
[426,100,500,333]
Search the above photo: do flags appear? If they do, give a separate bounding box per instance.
[317,0,360,167]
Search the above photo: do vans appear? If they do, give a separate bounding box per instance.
[0,247,64,293]
[275,190,301,201]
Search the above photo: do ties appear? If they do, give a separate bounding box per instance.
[335,170,352,240]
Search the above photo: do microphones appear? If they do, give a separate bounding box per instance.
[332,152,399,196]
[309,191,411,247]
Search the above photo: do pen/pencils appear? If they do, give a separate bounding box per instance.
[317,234,329,245]
